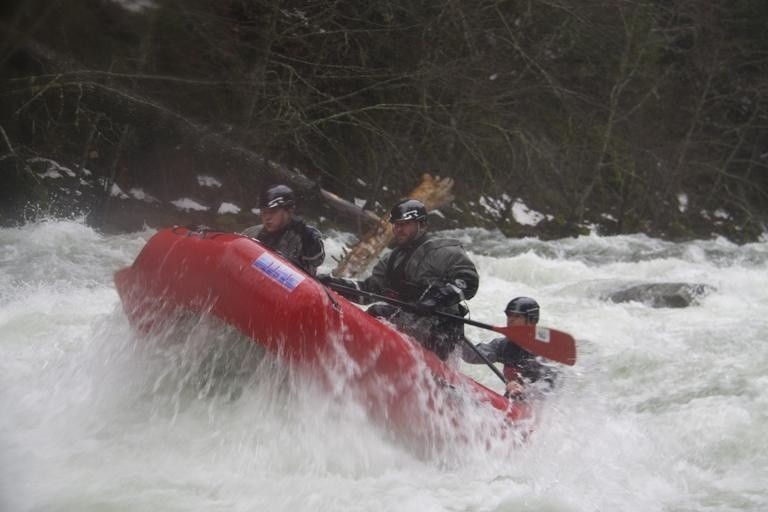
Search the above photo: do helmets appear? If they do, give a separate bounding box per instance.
[259,185,295,209]
[505,297,539,323]
[389,199,427,223]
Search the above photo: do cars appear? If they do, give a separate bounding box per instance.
[115,225,537,470]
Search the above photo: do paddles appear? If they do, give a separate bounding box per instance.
[328,282,576,366]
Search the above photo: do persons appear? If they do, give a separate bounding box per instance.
[462,296,558,402]
[239,184,325,279]
[318,199,480,362]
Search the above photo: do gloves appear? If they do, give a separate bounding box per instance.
[434,285,460,306]
[316,274,343,292]
